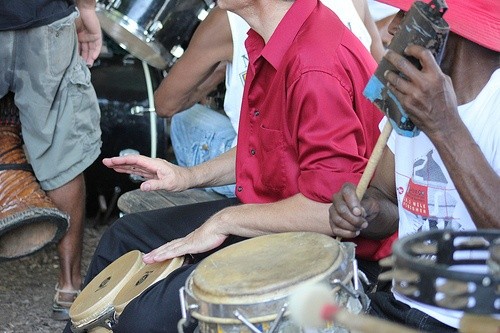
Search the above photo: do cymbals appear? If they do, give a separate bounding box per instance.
[391,225,500,316]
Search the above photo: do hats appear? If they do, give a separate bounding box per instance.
[373,0,500,54]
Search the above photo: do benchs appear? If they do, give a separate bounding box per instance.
[118,185,223,213]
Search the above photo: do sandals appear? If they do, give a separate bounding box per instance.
[48,281,83,322]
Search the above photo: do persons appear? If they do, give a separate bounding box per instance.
[63,0,398,333]
[0,0,103,319]
[329,0,500,333]
[153,0,386,199]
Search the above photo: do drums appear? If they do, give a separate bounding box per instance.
[0,97,72,263]
[93,0,211,73]
[64,249,190,332]
[178,228,370,333]
